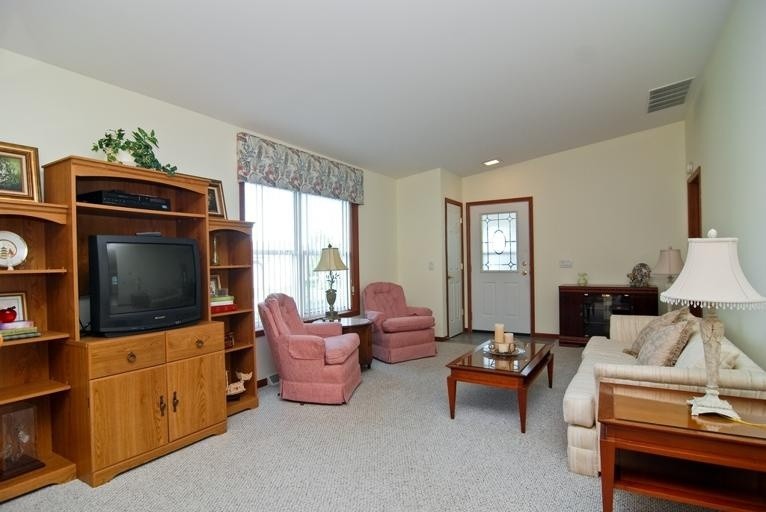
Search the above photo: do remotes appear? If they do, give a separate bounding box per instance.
[136,231,161,237]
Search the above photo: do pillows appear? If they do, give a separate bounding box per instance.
[634,320,697,372]
[622,307,684,360]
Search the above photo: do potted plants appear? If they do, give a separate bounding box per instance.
[88,125,178,178]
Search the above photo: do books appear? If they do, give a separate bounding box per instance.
[211,304,238,314]
[0,329,39,336]
[0,320,35,329]
[0,333,42,343]
[210,301,234,306]
[210,294,235,301]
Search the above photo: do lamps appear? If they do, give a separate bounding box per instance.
[312,243,353,321]
[658,225,765,423]
[652,247,694,322]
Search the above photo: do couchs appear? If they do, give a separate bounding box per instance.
[362,281,441,366]
[559,309,765,480]
[256,290,368,408]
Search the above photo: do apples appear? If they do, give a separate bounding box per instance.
[0,309,16,323]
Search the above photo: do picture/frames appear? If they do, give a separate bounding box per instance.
[1,139,44,206]
[0,291,29,321]
[208,179,228,219]
[208,272,221,297]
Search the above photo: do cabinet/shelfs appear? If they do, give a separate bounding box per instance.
[40,154,233,490]
[203,214,260,420]
[556,282,662,350]
[0,196,82,510]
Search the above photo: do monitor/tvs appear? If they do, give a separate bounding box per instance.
[88,235,201,338]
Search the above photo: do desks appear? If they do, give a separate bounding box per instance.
[594,375,766,512]
[309,314,374,374]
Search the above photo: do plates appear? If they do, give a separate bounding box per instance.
[0,230,29,268]
[632,262,651,281]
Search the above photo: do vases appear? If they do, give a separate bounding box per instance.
[575,273,591,288]
